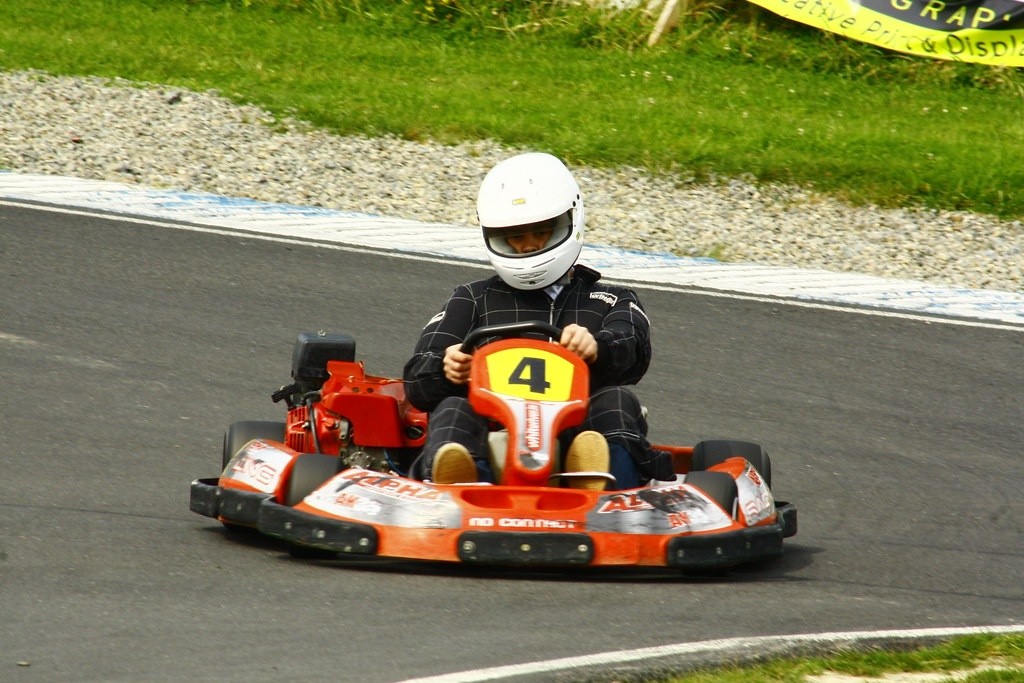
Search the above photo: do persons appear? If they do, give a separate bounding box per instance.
[400,150,654,491]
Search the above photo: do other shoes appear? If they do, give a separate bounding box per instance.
[432,442,477,485]
[565,430,609,492]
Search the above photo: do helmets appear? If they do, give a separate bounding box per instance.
[477,152,584,290]
